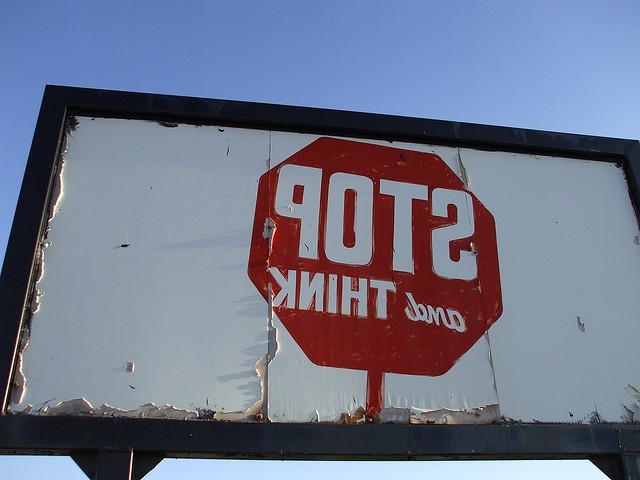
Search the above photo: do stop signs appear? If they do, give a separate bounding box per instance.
[245,133,503,380]
